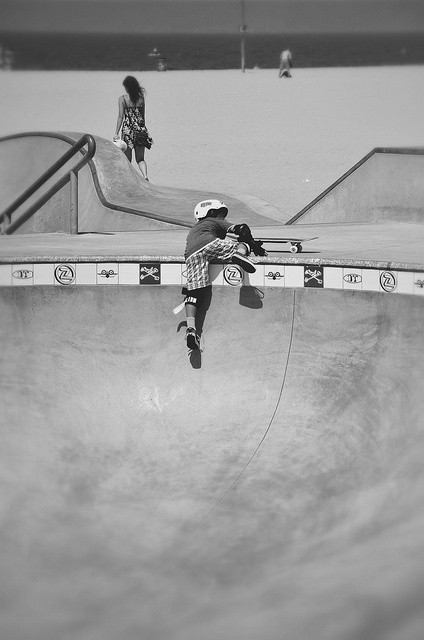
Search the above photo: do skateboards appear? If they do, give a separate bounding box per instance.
[226,235,319,253]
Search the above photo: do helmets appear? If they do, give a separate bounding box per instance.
[194,198,228,220]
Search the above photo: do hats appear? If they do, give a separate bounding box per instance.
[113,140,128,153]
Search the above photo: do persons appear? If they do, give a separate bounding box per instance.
[184,199,256,351]
[112,76,152,182]
[279,47,291,77]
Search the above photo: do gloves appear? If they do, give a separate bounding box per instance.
[254,247,268,258]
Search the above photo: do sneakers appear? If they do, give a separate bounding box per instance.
[185,327,200,354]
[231,252,256,275]
